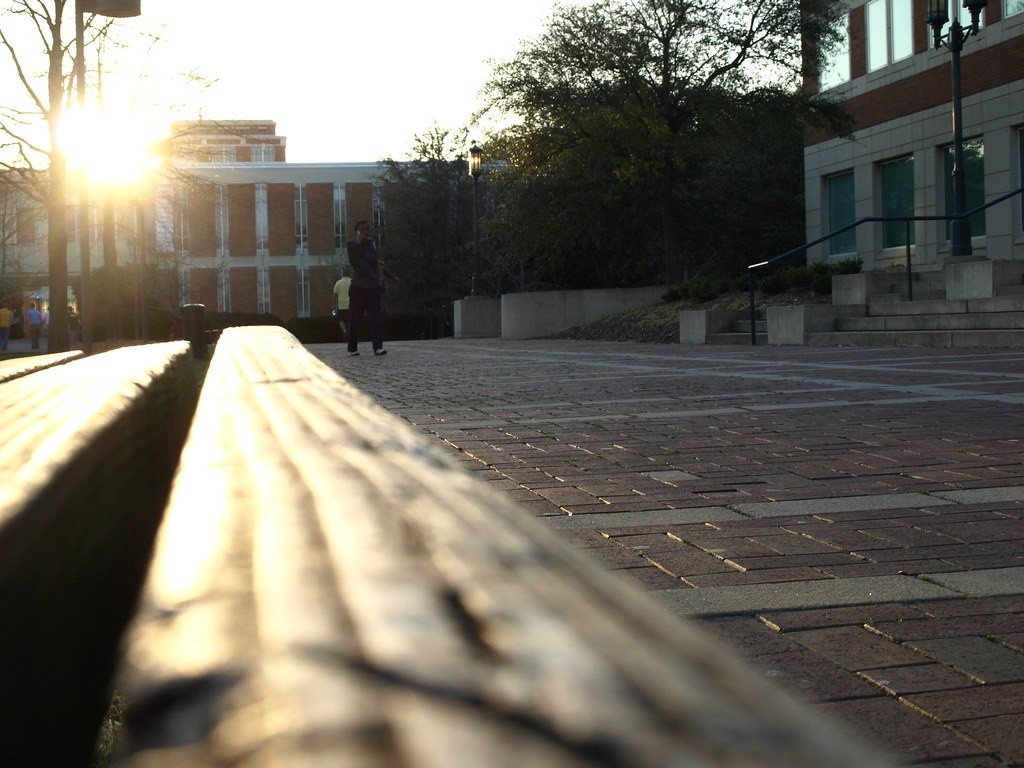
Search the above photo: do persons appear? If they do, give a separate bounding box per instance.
[347,220,387,355]
[12,309,20,337]
[44,306,49,327]
[24,303,42,349]
[67,307,82,347]
[330,263,352,342]
[0,302,13,352]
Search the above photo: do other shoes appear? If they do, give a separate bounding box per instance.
[343,332,348,342]
[374,348,386,355]
[349,351,360,356]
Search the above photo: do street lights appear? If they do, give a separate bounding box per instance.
[469,145,486,297]
[929,0,983,259]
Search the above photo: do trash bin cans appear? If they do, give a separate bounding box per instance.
[179,303,208,359]
